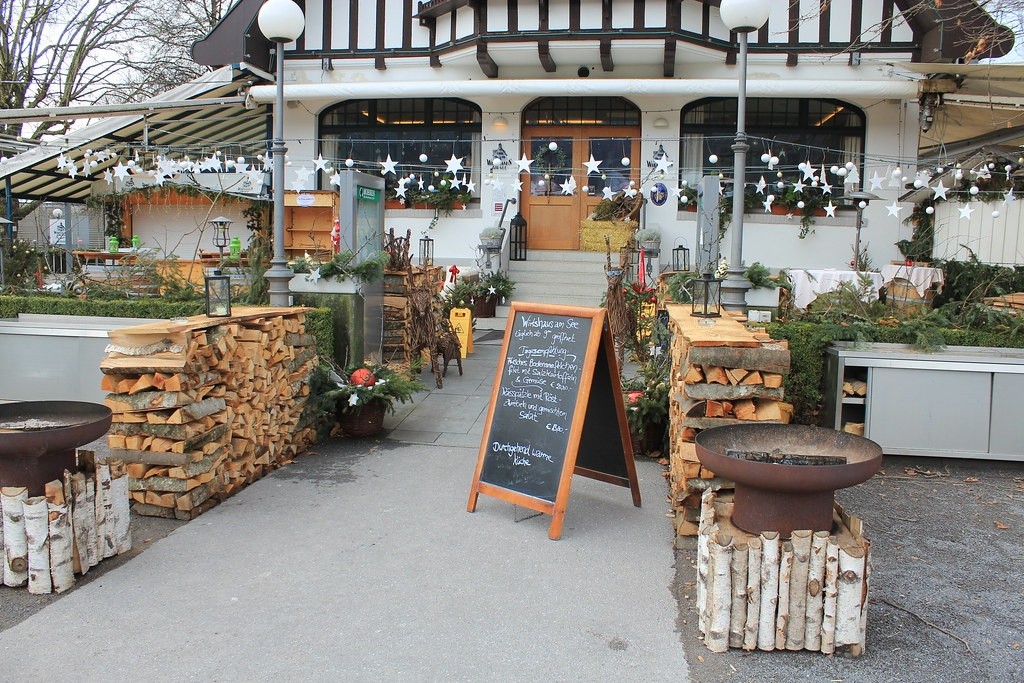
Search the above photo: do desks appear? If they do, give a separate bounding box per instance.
[72,251,136,267]
[784,270,885,309]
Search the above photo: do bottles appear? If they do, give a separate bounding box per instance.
[109,237,119,252]
[230,235,240,258]
[132,235,140,248]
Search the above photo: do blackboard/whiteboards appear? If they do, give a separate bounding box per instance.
[473,299,605,516]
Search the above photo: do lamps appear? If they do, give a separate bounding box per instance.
[690,271,723,318]
[653,118,669,127]
[510,212,528,260]
[206,271,231,318]
[836,192,887,269]
[419,236,434,266]
[672,244,690,272]
[0,217,15,285]
[493,116,508,126]
[208,216,235,259]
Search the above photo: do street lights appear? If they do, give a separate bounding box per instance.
[720,0,771,313]
[257,0,305,307]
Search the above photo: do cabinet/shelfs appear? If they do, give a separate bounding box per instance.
[824,341,1024,462]
[283,189,339,262]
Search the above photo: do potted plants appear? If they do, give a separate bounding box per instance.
[764,191,839,241]
[685,187,762,215]
[617,281,670,456]
[311,356,431,438]
[446,272,516,320]
[635,229,661,258]
[413,186,472,218]
[479,226,502,248]
[385,189,416,209]
[744,262,793,307]
